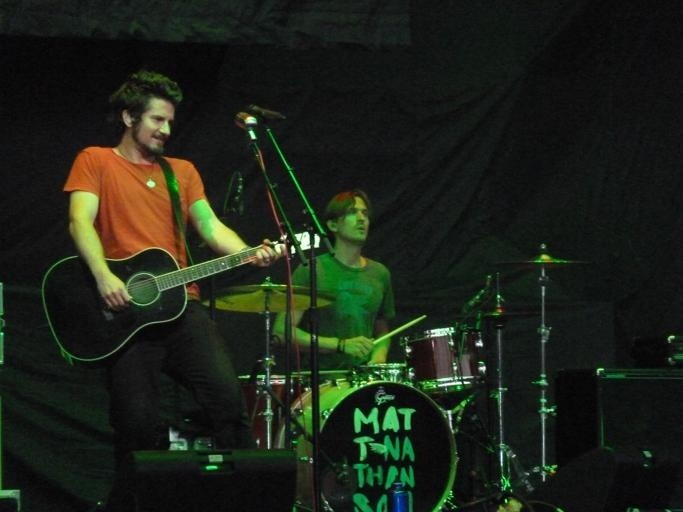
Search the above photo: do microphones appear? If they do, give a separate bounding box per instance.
[249,103,287,121]
[233,111,257,132]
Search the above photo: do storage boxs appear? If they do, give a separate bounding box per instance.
[554,360,683,464]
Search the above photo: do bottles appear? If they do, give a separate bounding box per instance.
[388,477,409,512]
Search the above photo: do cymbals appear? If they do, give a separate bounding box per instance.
[450,265,539,295]
[490,253,592,266]
[199,285,337,314]
[450,308,541,319]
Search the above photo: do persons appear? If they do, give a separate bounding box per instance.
[63,66,286,451]
[271,186,396,367]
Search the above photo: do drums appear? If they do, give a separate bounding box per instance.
[273,377,459,511]
[399,323,484,397]
[238,363,405,448]
[419,375,502,511]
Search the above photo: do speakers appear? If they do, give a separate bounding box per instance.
[558,368,683,512]
[102,447,297,512]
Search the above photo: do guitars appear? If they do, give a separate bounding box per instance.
[39,231,322,364]
